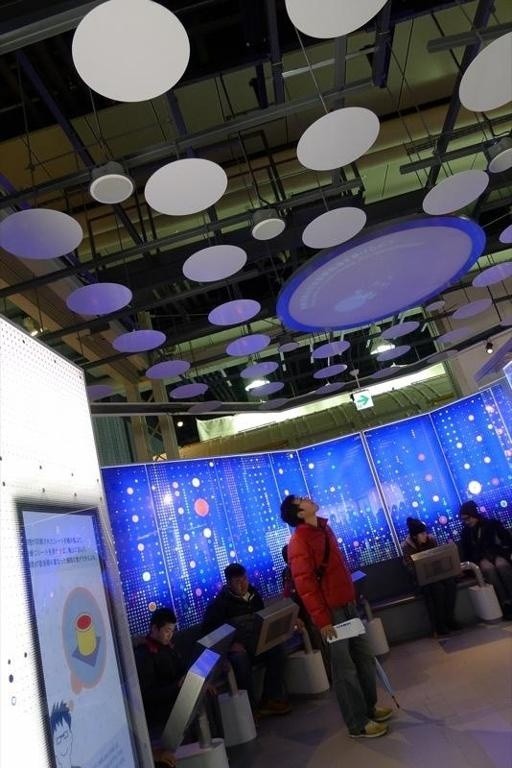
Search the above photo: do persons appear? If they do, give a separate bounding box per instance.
[281,493,395,740]
[459,498,512,622]
[150,744,179,767]
[278,543,333,685]
[49,700,82,768]
[134,606,230,762]
[201,562,292,716]
[400,516,465,643]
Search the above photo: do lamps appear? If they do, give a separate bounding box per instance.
[250,207,288,244]
[242,360,270,394]
[422,293,448,314]
[87,159,135,207]
[22,316,38,337]
[368,320,395,357]
[277,333,300,355]
[485,342,494,355]
[481,131,512,176]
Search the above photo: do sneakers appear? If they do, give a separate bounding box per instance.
[258,701,290,716]
[373,708,392,721]
[350,719,389,738]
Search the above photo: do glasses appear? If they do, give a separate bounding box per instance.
[460,518,470,521]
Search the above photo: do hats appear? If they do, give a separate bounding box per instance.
[406,516,426,535]
[460,501,478,517]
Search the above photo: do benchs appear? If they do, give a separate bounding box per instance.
[360,573,482,647]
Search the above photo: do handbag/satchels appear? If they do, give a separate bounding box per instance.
[288,570,325,608]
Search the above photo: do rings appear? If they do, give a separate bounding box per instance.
[301,623,303,626]
